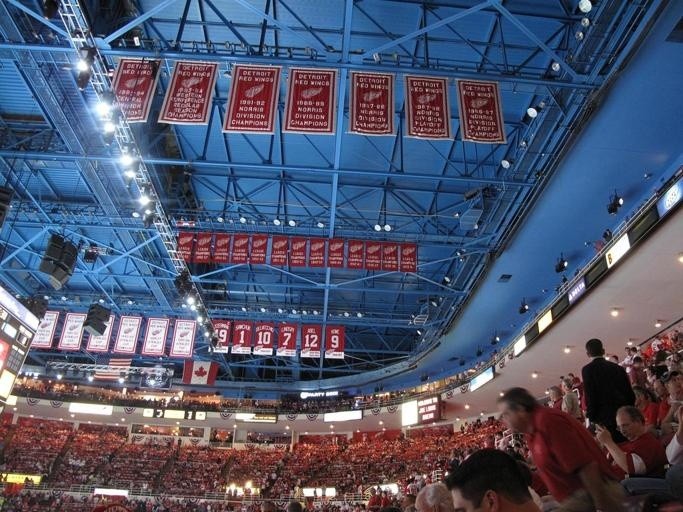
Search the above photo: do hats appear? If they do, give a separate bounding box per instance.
[660,371,678,382]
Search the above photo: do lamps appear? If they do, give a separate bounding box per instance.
[212,211,393,235]
[238,304,363,321]
[16,294,135,305]
[492,0,595,176]
[74,44,217,351]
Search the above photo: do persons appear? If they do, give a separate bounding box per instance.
[1,179,683,511]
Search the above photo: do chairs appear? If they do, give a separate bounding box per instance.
[0,419,479,512]
